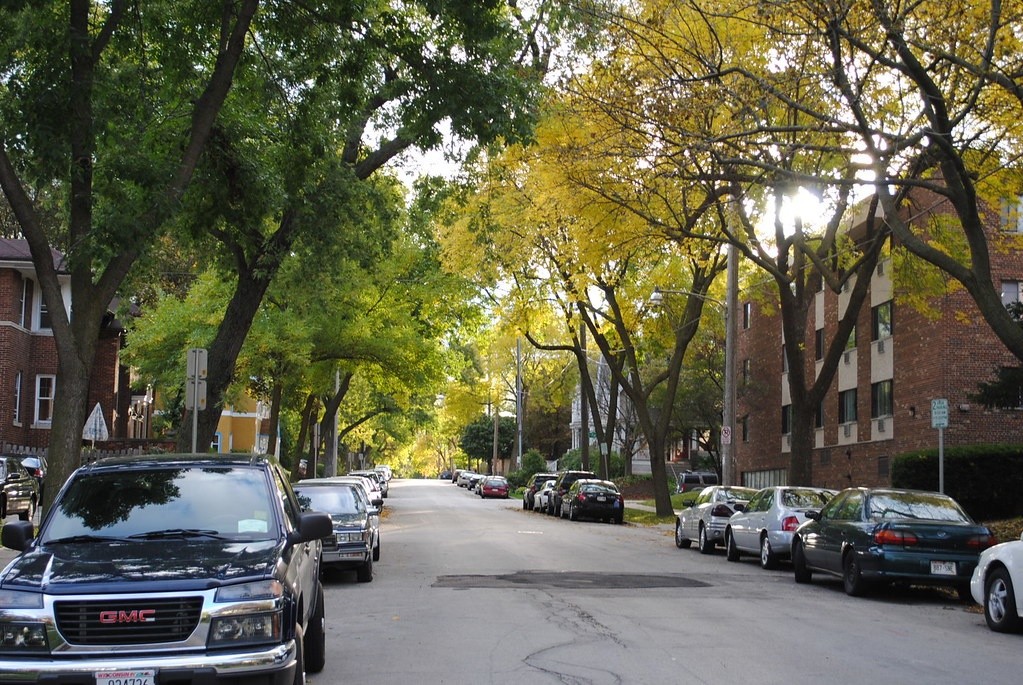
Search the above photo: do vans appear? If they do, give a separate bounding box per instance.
[678,470,720,494]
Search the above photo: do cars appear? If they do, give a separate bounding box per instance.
[1,456,40,521]
[0,452,335,685]
[532,479,557,514]
[452,469,487,496]
[440,470,453,480]
[297,465,394,558]
[481,478,509,499]
[970,533,1023,632]
[674,485,762,554]
[285,483,384,582]
[791,487,998,606]
[560,479,624,525]
[10,454,48,491]
[724,485,838,571]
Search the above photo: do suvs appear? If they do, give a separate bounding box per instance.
[548,471,599,517]
[521,473,558,511]
